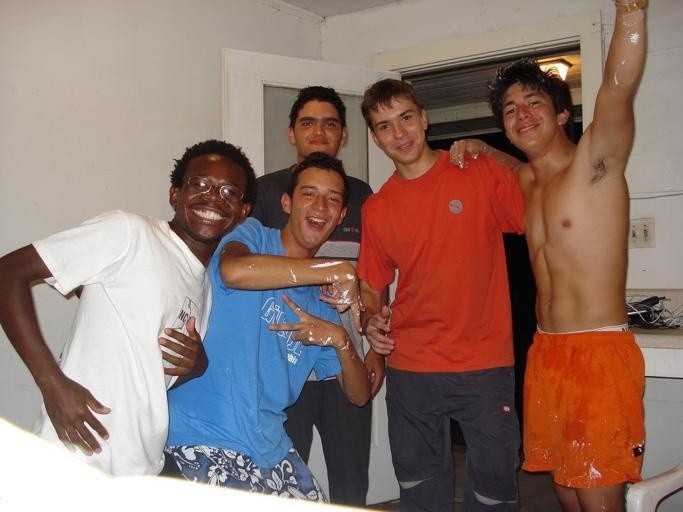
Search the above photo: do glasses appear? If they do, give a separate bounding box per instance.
[183,176,245,202]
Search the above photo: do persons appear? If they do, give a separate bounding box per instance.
[448,0,648,512]
[356,80,525,512]
[0,139,260,478]
[248,86,373,507]
[164,153,373,503]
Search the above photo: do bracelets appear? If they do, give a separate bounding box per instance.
[615,0,648,13]
